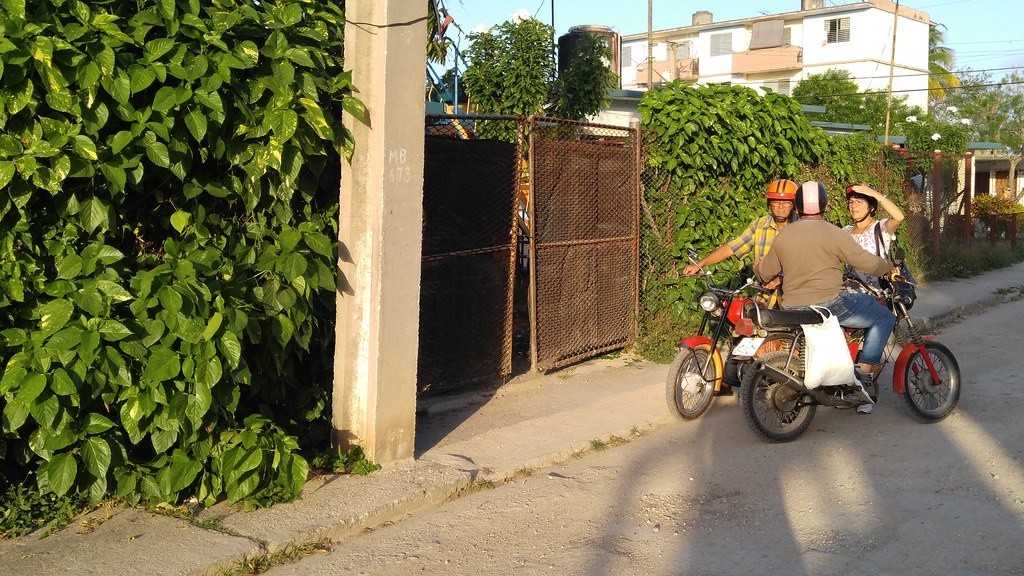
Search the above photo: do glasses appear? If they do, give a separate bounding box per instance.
[846,199,866,206]
[770,201,793,208]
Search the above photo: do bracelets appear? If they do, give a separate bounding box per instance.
[880,195,887,202]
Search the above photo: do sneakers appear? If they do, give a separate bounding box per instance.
[851,363,877,403]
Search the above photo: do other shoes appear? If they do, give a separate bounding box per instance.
[713,384,733,396]
[856,404,874,414]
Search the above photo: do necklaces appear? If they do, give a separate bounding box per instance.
[856,219,873,243]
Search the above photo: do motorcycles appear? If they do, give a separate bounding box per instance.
[727,246,961,444]
[662,247,784,423]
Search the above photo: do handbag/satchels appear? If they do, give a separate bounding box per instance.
[873,222,917,311]
[800,305,854,390]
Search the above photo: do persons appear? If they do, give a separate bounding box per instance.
[833,184,905,413]
[755,180,901,406]
[682,179,798,396]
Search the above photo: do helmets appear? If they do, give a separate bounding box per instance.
[846,184,877,211]
[765,179,798,210]
[794,181,827,215]
